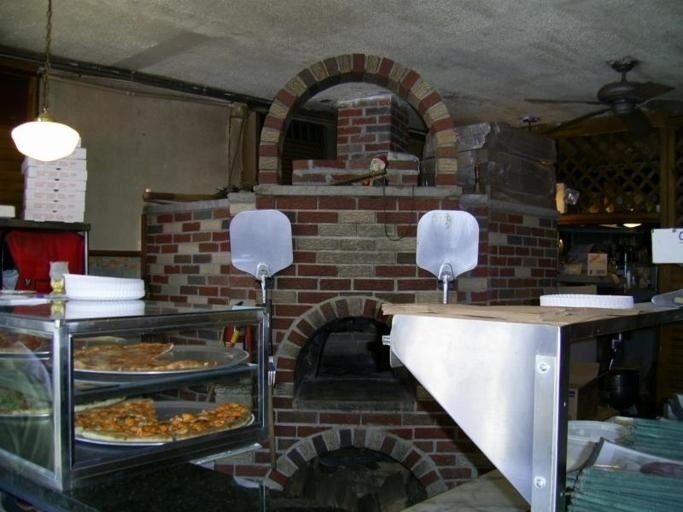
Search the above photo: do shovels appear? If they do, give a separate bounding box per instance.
[416,210,480,303]
[229,210,293,304]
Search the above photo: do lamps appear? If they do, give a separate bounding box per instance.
[10,0,78,163]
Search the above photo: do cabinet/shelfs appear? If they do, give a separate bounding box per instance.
[0,285,273,487]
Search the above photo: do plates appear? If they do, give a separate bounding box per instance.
[71,401,257,450]
[0,289,37,301]
[71,345,250,377]
[567,418,626,443]
[1,394,126,421]
[0,336,124,356]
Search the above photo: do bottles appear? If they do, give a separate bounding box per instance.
[587,189,641,216]
[620,238,634,267]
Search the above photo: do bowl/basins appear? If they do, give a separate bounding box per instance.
[609,368,643,411]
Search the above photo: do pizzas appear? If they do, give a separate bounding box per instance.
[74,397,252,442]
[0,330,50,353]
[72,343,216,372]
[1,387,126,415]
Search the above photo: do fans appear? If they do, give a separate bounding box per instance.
[517,50,677,143]
